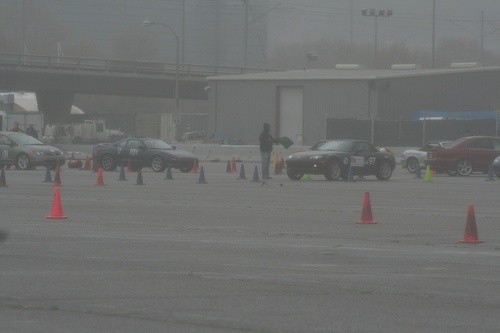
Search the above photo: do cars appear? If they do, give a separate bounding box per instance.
[400,140,456,173]
[423,136,500,177]
[93,137,200,173]
[0,131,66,171]
[285,138,396,182]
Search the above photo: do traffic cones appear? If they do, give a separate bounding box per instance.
[51,167,64,185]
[196,165,208,184]
[190,159,199,174]
[225,155,238,173]
[45,186,68,218]
[248,165,260,183]
[164,163,174,180]
[95,167,107,186]
[484,163,496,181]
[422,164,435,183]
[81,156,93,171]
[117,167,128,181]
[238,164,248,180]
[301,173,312,182]
[352,191,378,224]
[43,164,54,183]
[54,159,63,173]
[135,170,146,185]
[456,203,485,244]
[0,169,8,187]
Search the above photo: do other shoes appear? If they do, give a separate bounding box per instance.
[262,176,272,179]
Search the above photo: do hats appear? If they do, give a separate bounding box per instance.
[263,122,270,127]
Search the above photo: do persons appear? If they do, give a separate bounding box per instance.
[11,121,24,133]
[25,122,39,140]
[259,123,279,180]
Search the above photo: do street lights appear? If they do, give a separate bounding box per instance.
[360,8,394,144]
[142,19,180,148]
